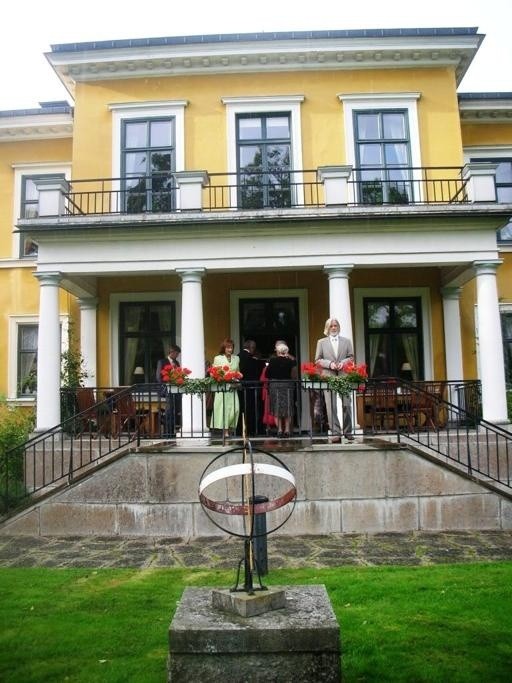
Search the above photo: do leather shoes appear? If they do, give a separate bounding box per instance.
[344,434,355,440]
[331,437,341,443]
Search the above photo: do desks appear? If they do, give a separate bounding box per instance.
[112,409,160,438]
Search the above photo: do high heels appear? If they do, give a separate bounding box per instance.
[278,431,290,438]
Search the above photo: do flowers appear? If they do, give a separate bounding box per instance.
[342,361,369,383]
[207,366,243,382]
[160,365,192,384]
[301,362,321,380]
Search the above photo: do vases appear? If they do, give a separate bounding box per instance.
[303,381,366,390]
[166,383,229,394]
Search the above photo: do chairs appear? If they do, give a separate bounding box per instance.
[423,380,448,430]
[71,388,111,439]
[373,379,402,434]
[356,396,379,435]
[102,390,146,440]
[403,381,437,434]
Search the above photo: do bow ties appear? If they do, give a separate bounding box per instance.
[332,338,338,342]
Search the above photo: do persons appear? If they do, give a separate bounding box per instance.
[156,345,181,439]
[275,341,296,382]
[210,339,240,438]
[315,318,355,443]
[237,340,267,436]
[264,344,297,438]
